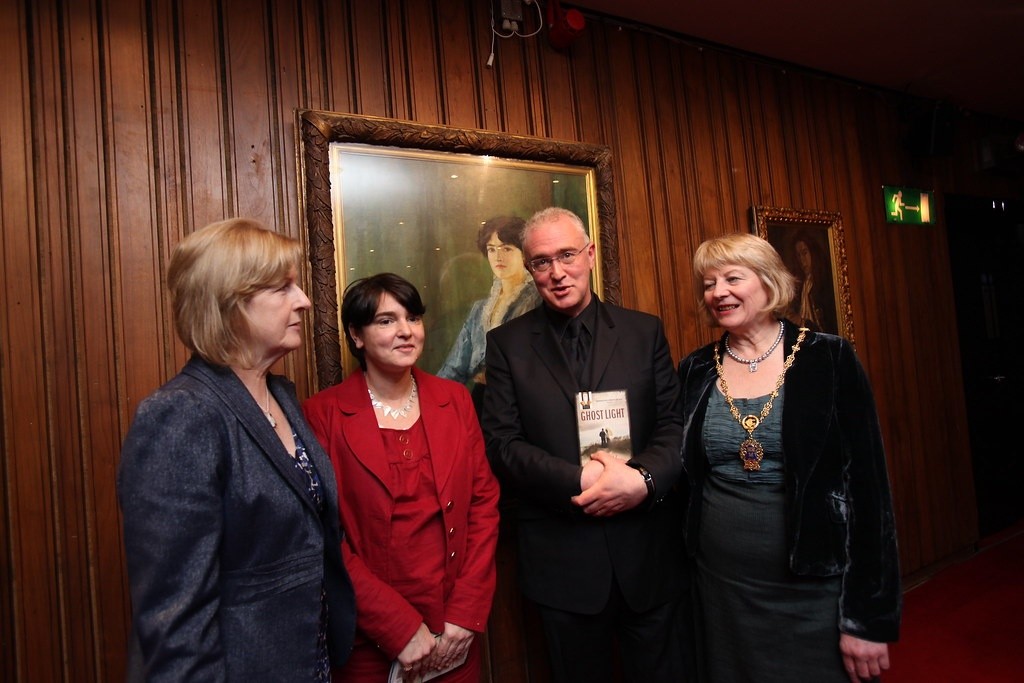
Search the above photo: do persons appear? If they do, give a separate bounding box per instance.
[676,233,904,683]
[115,216,358,683]
[599,428,611,445]
[484,207,685,683]
[300,273,501,683]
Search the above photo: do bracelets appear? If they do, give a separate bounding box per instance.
[640,468,654,495]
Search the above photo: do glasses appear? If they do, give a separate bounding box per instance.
[524,242,590,272]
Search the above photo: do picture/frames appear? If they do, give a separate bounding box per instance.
[746,204,857,351]
[294,109,624,396]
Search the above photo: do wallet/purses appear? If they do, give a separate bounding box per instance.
[388,632,469,683]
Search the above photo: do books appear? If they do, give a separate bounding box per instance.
[575,389,634,466]
[388,633,468,683]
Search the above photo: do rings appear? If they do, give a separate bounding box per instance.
[403,667,413,672]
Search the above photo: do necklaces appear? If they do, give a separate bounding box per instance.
[256,388,277,428]
[725,321,784,372]
[369,375,417,419]
[713,328,811,470]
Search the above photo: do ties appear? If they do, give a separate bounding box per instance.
[565,319,587,388]
